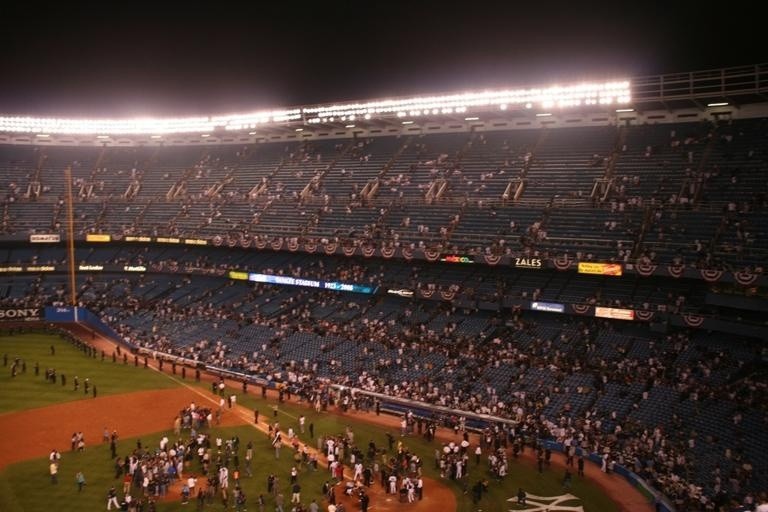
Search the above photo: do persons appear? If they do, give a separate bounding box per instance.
[0,115,768,511]
